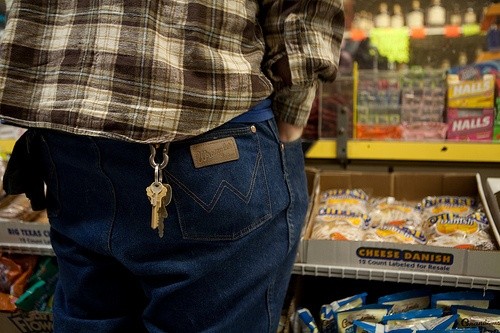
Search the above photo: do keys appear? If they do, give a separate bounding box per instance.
[146,181,172,237]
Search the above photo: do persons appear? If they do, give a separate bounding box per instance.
[1,0,346,333]
